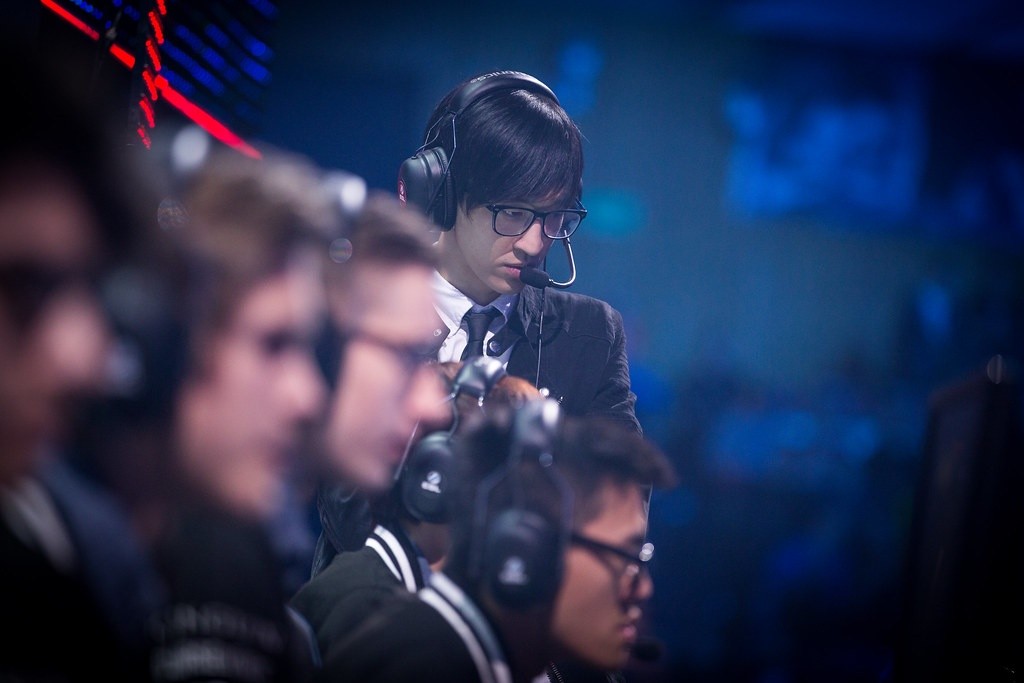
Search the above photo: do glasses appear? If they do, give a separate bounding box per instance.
[569,533,655,578]
[355,328,452,381]
[486,197,587,240]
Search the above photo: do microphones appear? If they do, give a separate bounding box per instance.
[519,228,576,289]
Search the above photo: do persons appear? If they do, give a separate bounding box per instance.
[0,142,674,683]
[311,71,643,581]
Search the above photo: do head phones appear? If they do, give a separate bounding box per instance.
[95,125,562,616]
[399,70,562,230]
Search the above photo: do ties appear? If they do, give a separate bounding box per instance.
[459,305,503,361]
